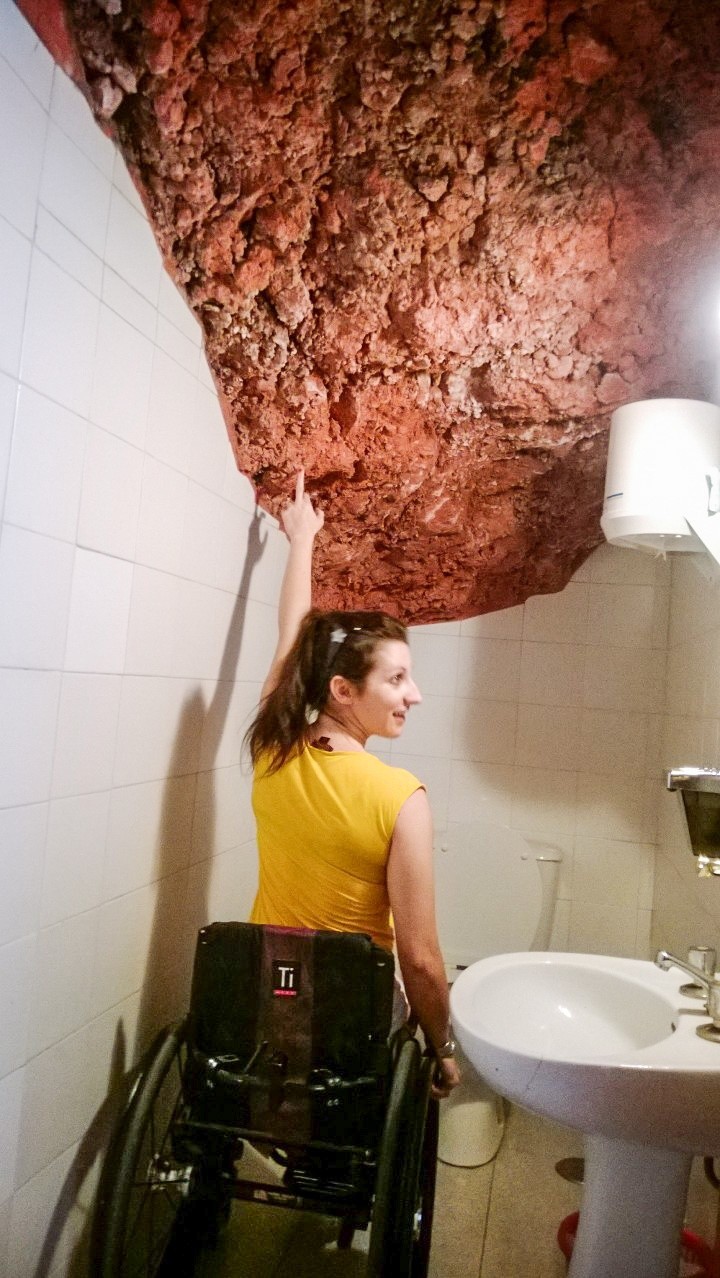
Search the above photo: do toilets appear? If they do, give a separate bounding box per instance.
[412,821,565,1171]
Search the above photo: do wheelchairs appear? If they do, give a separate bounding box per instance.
[88,918,467,1278]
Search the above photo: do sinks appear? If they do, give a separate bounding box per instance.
[444,948,720,1144]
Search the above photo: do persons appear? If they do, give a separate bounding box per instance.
[242,464,461,1103]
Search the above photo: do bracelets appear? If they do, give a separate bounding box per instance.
[435,1040,457,1059]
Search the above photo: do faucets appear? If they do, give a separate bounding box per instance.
[653,948,720,1043]
